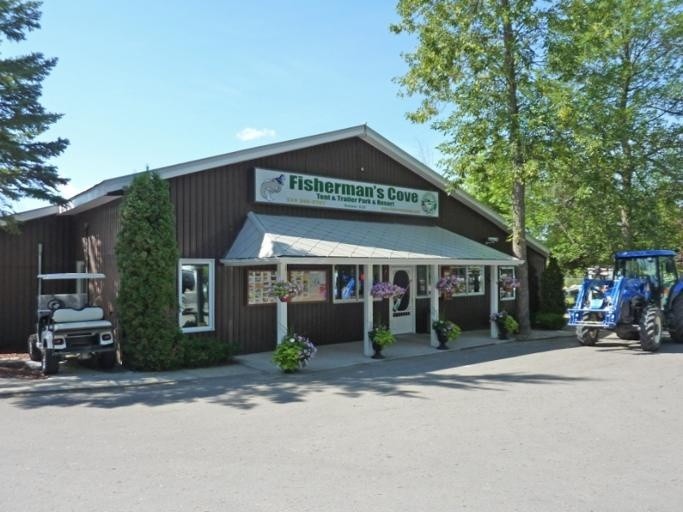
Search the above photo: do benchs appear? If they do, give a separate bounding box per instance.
[51,305,104,322]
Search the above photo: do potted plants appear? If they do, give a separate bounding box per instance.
[368,325,396,359]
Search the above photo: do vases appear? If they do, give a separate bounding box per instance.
[279,294,290,302]
[436,335,450,349]
[503,286,512,291]
[500,330,513,339]
[444,290,453,301]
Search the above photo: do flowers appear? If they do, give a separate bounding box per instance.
[271,280,305,297]
[369,281,405,298]
[435,275,461,293]
[431,319,461,340]
[273,332,317,371]
[488,310,519,330]
[498,276,520,289]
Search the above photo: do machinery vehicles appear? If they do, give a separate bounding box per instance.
[566,246,683,354]
